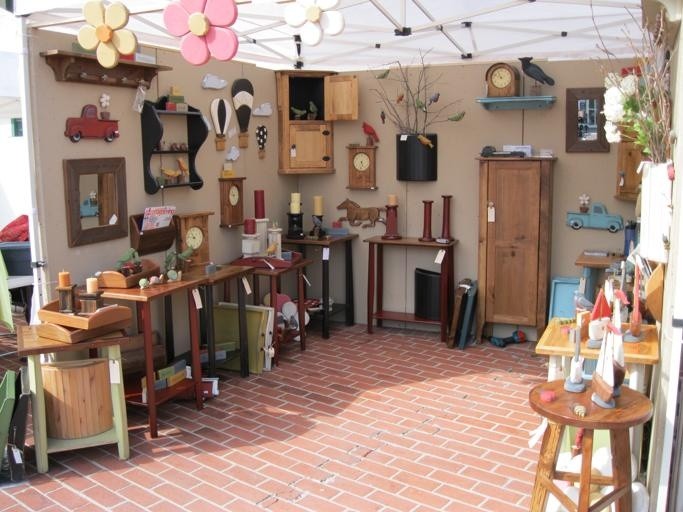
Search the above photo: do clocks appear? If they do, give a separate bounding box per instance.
[219,175,248,228]
[346,144,379,192]
[486,63,520,98]
[173,210,215,268]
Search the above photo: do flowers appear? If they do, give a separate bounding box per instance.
[371,49,465,131]
[587,0,673,162]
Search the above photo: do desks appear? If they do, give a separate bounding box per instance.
[15,323,136,476]
[537,315,659,475]
[280,233,360,339]
[75,268,204,440]
[176,261,254,383]
[226,260,313,365]
[364,232,459,342]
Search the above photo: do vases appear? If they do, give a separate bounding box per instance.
[396,132,438,183]
[636,163,670,266]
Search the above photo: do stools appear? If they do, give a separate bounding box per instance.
[529,378,653,512]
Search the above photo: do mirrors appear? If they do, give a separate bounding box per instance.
[64,157,129,246]
[565,87,611,153]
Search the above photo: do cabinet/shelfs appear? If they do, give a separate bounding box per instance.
[275,71,357,168]
[472,154,558,347]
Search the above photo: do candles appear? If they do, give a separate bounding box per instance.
[85,272,96,293]
[245,214,256,235]
[312,195,322,216]
[58,268,70,288]
[291,192,302,214]
[254,187,266,219]
[387,193,395,207]
